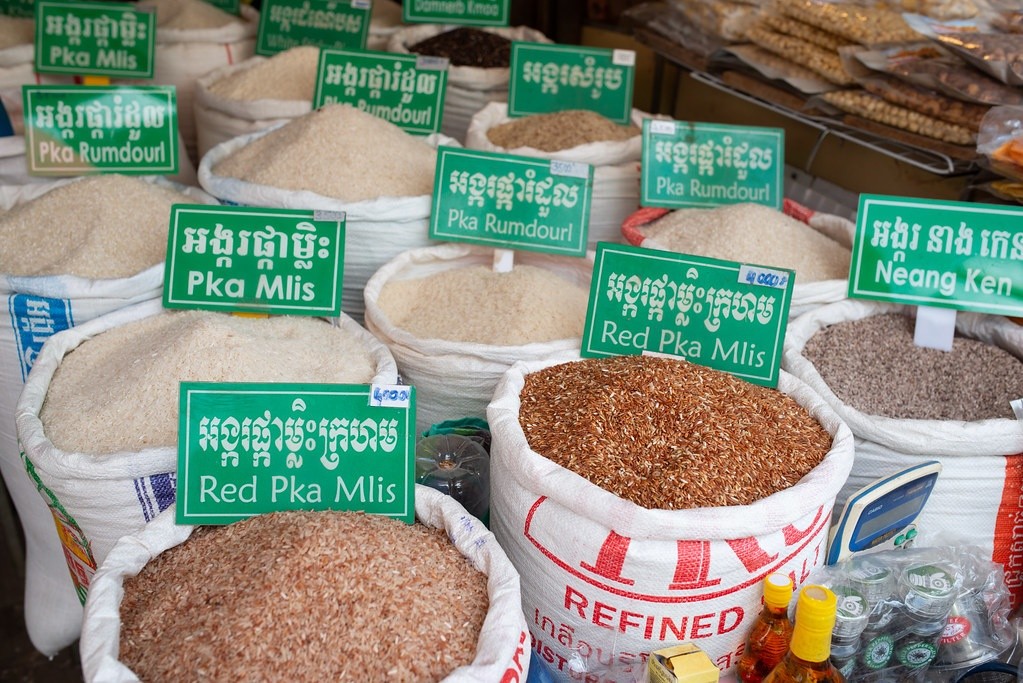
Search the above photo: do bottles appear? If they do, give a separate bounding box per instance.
[755,585,848,683]
[832,555,958,682]
[414,432,490,520]
[735,573,793,683]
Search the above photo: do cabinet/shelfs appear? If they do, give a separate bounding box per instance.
[585,25,978,203]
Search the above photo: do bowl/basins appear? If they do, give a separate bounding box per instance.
[929,582,998,660]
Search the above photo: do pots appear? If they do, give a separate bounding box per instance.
[924,626,1023,683]
